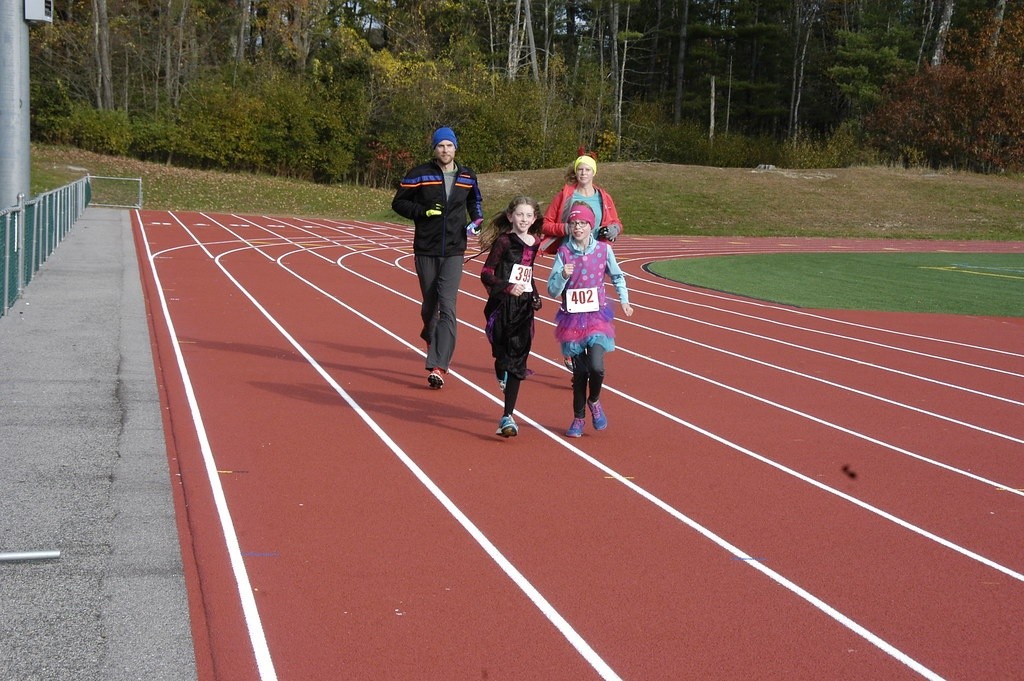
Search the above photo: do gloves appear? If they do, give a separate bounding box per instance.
[603,224,620,241]
[423,203,443,218]
[471,225,482,237]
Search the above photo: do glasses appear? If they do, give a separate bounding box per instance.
[569,220,589,229]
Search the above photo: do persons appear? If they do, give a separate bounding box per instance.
[391,128,483,389]
[541,146,624,255]
[547,201,634,437]
[478,196,543,439]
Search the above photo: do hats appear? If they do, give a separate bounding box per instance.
[432,127,458,151]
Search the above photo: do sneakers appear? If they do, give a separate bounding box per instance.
[498,371,507,393]
[567,418,584,438]
[564,353,574,370]
[426,342,430,352]
[428,367,444,390]
[496,414,518,438]
[587,398,607,431]
